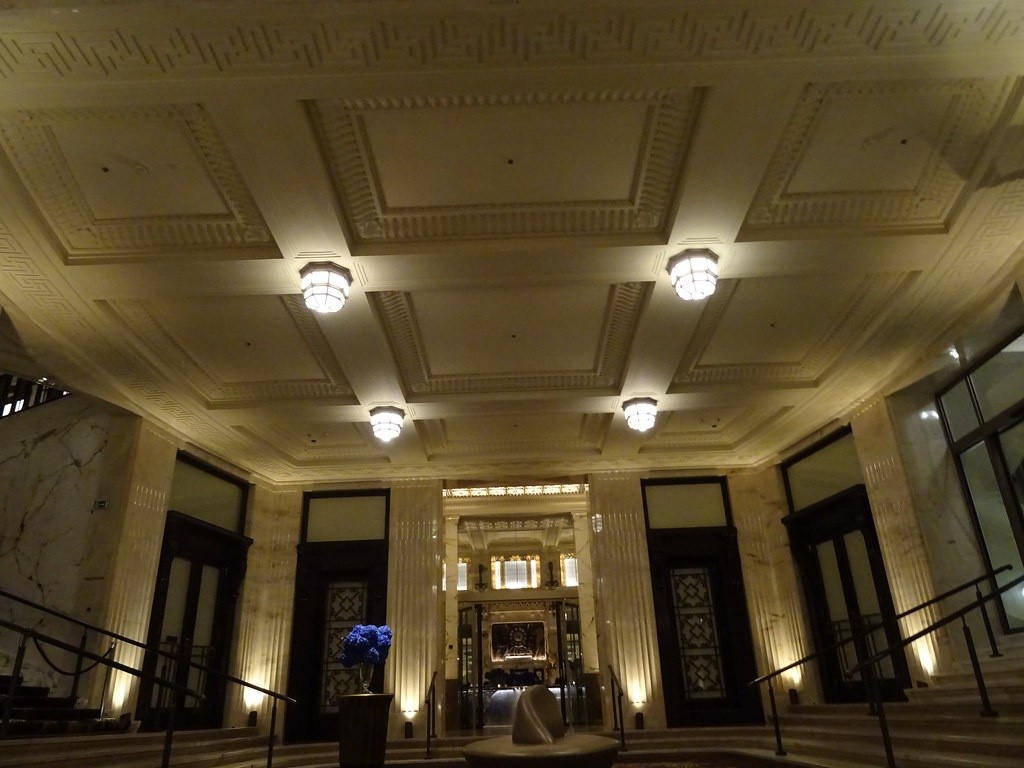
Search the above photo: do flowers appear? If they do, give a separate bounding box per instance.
[340,621,393,694]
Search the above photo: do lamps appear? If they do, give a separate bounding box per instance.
[248,711,258,727]
[369,406,405,443]
[916,681,928,688]
[298,260,353,313]
[665,249,719,301]
[622,397,658,433]
[405,722,413,739]
[635,712,644,730]
[789,689,800,705]
[119,712,132,733]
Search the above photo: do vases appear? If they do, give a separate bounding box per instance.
[338,693,395,768]
